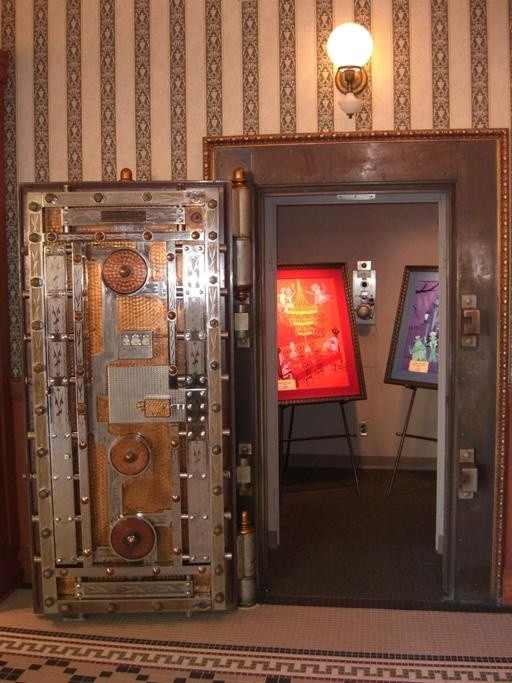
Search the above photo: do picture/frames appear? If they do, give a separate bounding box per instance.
[277,261,367,407]
[383,265,439,390]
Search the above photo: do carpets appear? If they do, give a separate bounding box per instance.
[1,579,511,683]
